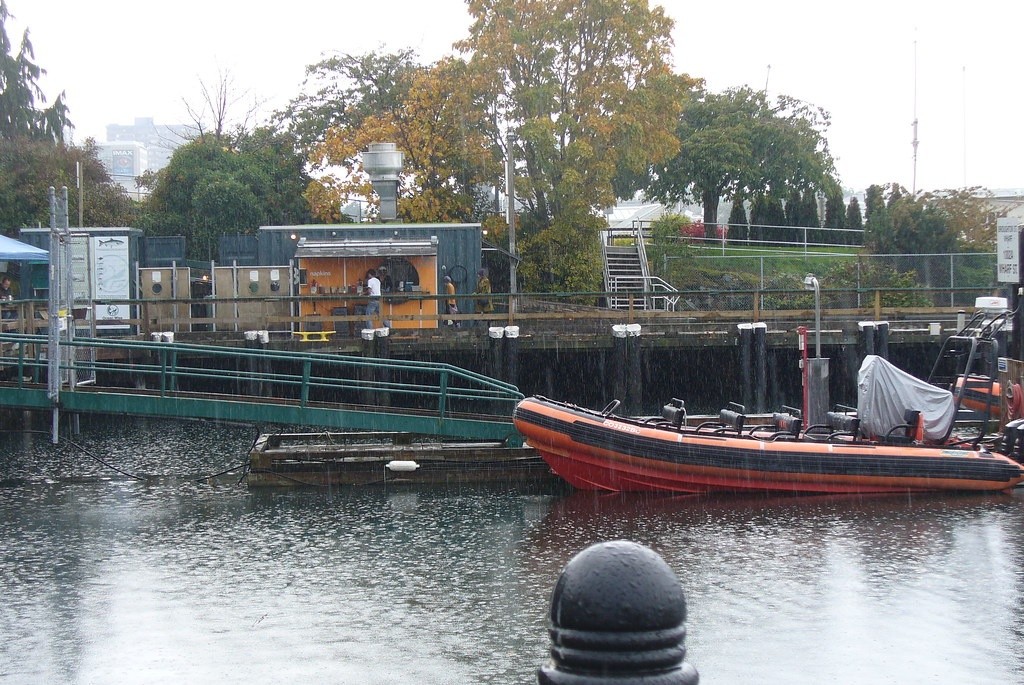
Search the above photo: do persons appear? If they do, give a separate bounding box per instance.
[361,269,392,329]
[375,266,393,292]
[0,276,19,319]
[443,276,461,329]
[472,268,493,334]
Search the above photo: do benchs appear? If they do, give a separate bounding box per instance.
[696,401,746,439]
[645,397,685,432]
[873,409,921,447]
[743,405,801,442]
[802,403,861,444]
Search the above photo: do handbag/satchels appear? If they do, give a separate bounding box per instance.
[478,299,489,306]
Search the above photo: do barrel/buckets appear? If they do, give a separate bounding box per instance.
[302,313,322,337]
[353,304,366,336]
[332,307,350,337]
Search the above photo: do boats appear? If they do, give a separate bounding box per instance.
[955,373,1001,414]
[513,392,1023,494]
